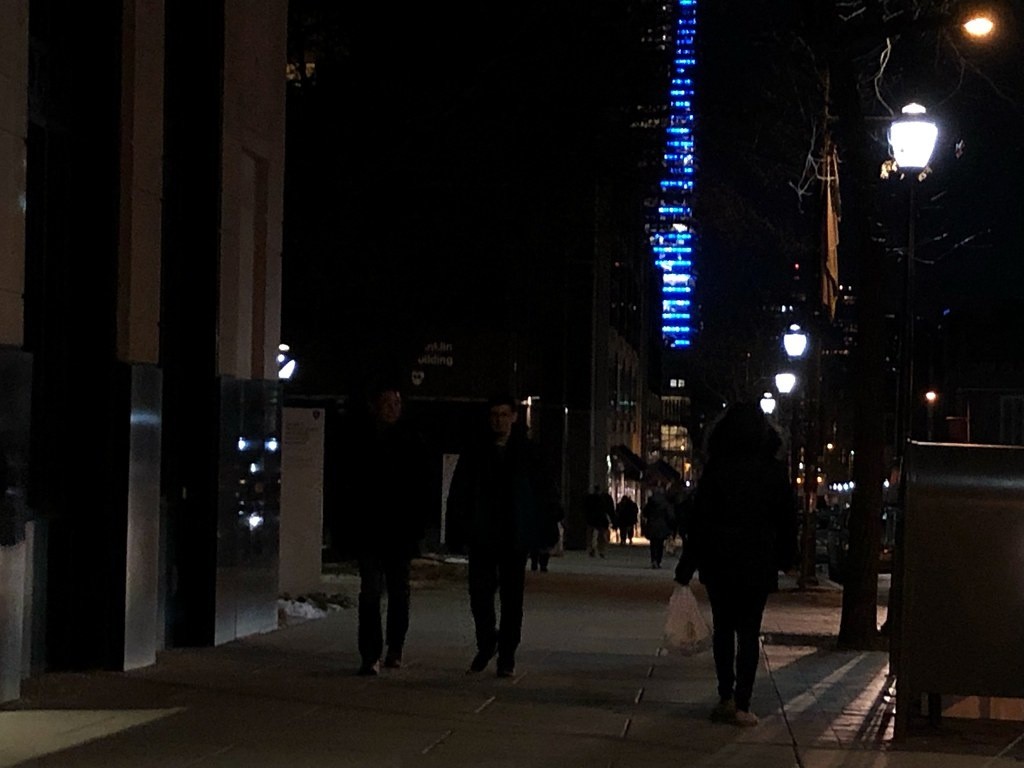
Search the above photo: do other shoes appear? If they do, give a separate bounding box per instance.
[496,668,515,677]
[360,660,379,675]
[471,628,499,672]
[714,700,735,716]
[735,710,759,725]
[385,657,400,667]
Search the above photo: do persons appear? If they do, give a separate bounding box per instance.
[642,490,676,569]
[673,404,801,725]
[617,494,638,548]
[325,386,441,678]
[444,389,559,675]
[584,483,619,558]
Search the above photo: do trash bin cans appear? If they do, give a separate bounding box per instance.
[886,436,1024,737]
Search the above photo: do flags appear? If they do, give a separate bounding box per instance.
[820,139,842,322]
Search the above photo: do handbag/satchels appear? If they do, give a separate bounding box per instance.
[659,582,713,656]
